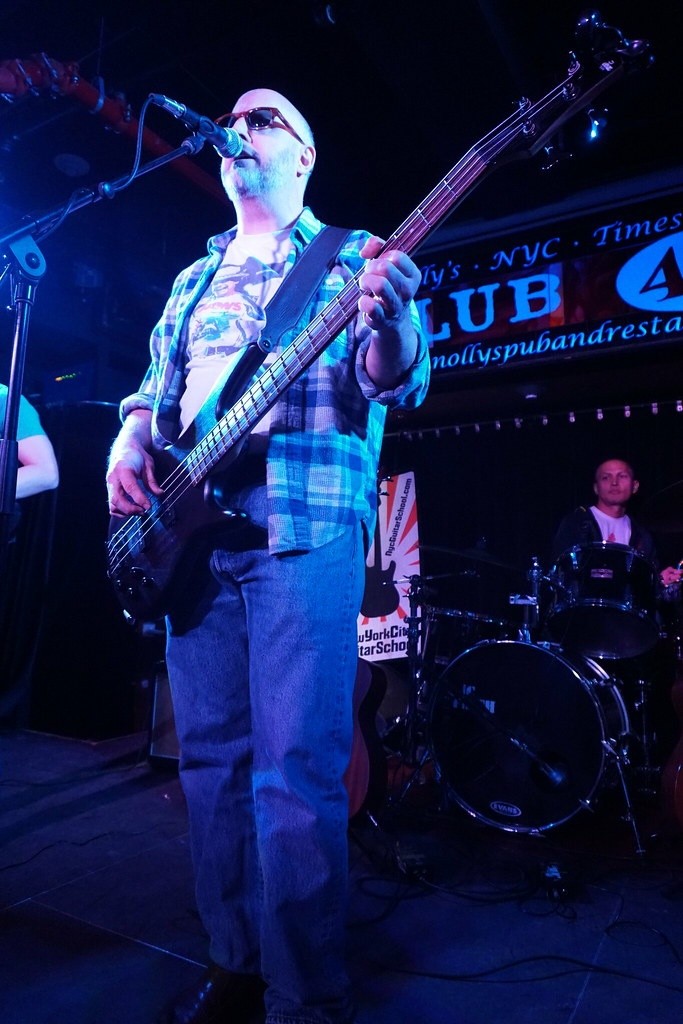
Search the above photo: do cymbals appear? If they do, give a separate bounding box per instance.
[419,535,528,575]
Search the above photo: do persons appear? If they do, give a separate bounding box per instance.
[0,383,60,500]
[550,459,683,595]
[103,88,429,1023]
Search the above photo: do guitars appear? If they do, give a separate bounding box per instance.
[102,11,657,629]
[342,656,389,830]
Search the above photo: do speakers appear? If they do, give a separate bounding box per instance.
[149,670,182,760]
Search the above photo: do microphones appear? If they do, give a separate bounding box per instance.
[546,768,568,789]
[148,93,244,158]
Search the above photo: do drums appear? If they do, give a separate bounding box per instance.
[425,638,631,838]
[547,540,665,660]
[411,602,517,712]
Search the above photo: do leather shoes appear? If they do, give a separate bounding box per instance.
[152,969,264,1024]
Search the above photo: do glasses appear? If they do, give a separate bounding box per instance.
[214,106,303,144]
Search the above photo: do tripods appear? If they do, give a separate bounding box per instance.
[376,561,480,769]
